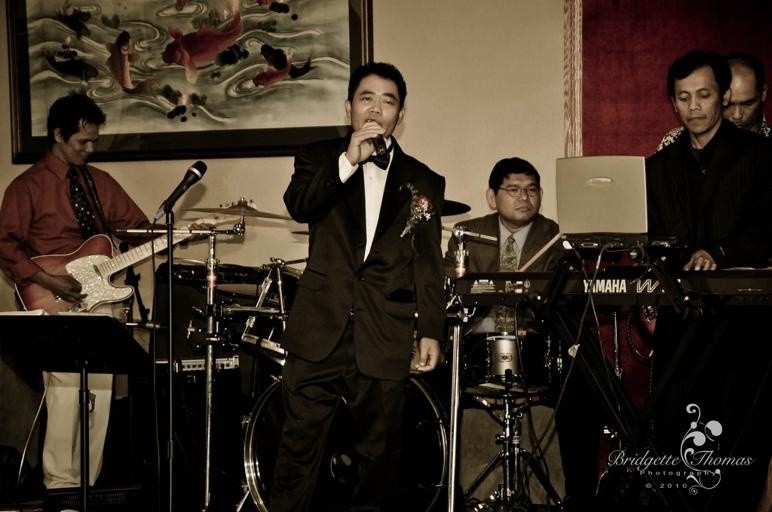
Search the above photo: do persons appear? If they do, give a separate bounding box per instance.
[443,154,606,508]
[644,47,772,512]
[265,60,449,512]
[653,51,772,155]
[1,84,218,495]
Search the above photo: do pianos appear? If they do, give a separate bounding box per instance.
[451,270,769,303]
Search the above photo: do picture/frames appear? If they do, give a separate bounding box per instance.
[4,1,373,166]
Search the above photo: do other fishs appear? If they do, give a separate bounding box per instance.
[105,30,157,95]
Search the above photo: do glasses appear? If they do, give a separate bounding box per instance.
[499,187,541,197]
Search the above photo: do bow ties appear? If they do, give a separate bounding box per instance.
[358,153,390,169]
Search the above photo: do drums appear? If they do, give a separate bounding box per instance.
[240,259,307,358]
[243,370,449,511]
[462,330,552,397]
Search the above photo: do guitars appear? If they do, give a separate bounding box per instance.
[15,216,224,314]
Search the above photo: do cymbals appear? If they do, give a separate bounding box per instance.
[181,206,294,221]
[442,200,470,216]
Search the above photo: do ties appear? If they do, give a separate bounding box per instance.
[66,168,96,239]
[494,236,518,333]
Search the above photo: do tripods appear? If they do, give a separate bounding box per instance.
[464,396,563,512]
[589,305,658,502]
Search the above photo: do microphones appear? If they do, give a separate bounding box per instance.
[155,160,207,221]
[372,135,390,162]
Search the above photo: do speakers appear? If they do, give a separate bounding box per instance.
[149,262,298,512]
